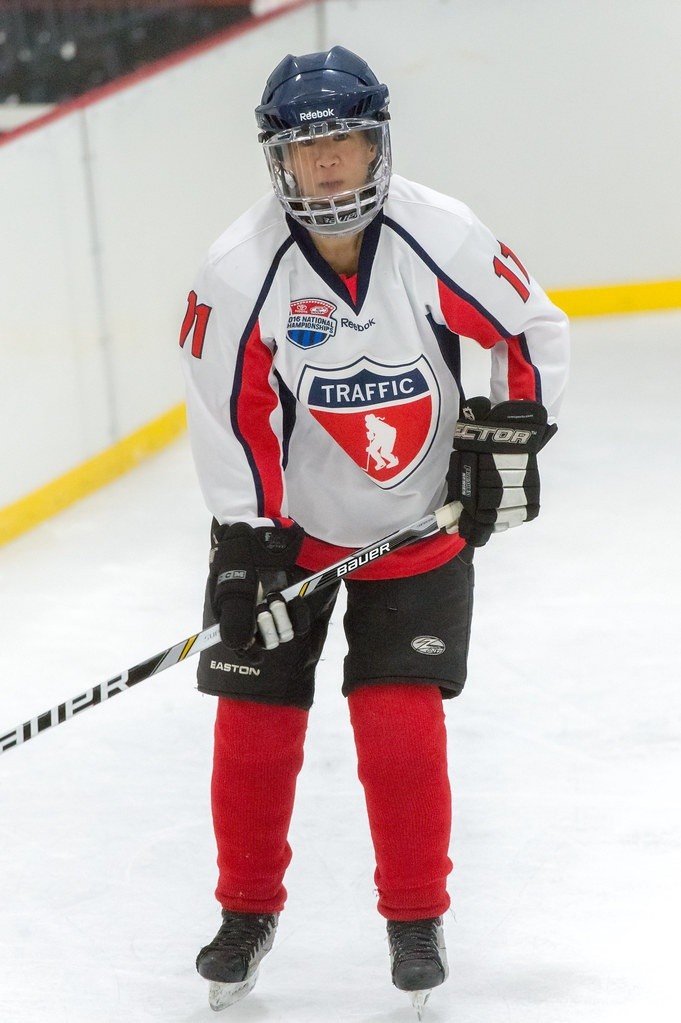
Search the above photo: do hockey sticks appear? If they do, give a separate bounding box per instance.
[0,499,471,756]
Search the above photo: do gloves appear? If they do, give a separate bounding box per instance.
[209,523,305,665]
[448,395,558,548]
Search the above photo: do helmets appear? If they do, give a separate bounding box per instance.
[256,46,391,237]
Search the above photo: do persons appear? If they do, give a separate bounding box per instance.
[165,42,569,995]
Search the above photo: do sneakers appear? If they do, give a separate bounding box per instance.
[195,909,280,1011]
[385,916,447,1020]
